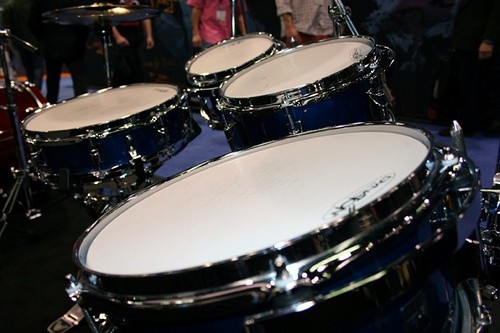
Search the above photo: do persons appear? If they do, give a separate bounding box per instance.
[275,0,336,47]
[373,0,454,117]
[439,0,500,137]
[0,0,189,104]
[185,0,248,51]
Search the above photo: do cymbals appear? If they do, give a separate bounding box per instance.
[35,3,162,27]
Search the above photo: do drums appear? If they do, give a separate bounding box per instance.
[485,172,500,332]
[217,35,396,151]
[0,80,52,227]
[21,83,202,207]
[184,33,288,131]
[72,119,482,333]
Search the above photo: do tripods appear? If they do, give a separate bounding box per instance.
[0,29,44,236]
